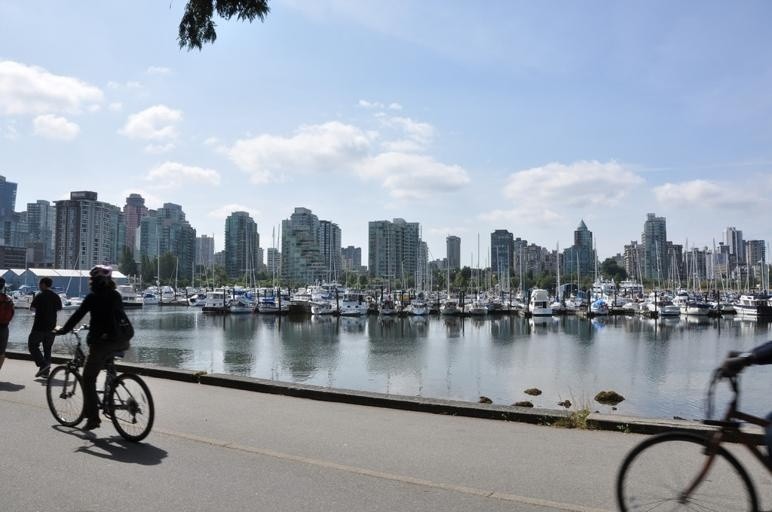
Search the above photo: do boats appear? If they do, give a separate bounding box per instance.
[524,315,772,342]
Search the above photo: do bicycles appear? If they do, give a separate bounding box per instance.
[613,344,771,511]
[40,323,156,444]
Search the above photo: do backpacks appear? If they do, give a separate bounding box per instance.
[96,294,133,342]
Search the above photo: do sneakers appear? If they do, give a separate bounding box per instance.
[36,364,50,378]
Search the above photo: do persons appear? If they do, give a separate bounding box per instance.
[722,340,772,457]
[53,265,135,431]
[29,277,62,377]
[0,277,15,369]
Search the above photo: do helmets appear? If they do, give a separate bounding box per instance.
[91,264,112,279]
